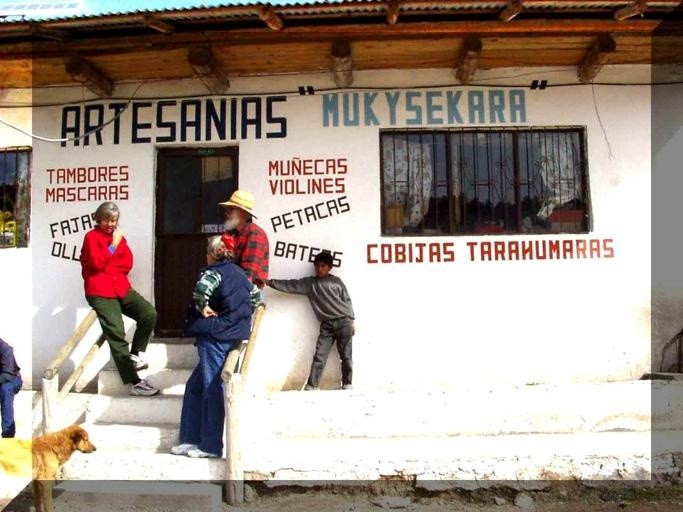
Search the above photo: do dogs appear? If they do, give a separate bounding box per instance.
[0,425,97,512]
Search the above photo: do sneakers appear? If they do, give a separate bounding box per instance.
[131,380,160,395]
[188,449,217,458]
[170,445,197,454]
[130,353,148,370]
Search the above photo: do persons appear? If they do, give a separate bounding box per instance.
[170,234,262,459]
[0,337,23,437]
[265,251,356,390]
[217,190,269,309]
[79,201,160,395]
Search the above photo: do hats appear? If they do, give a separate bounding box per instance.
[219,191,257,218]
